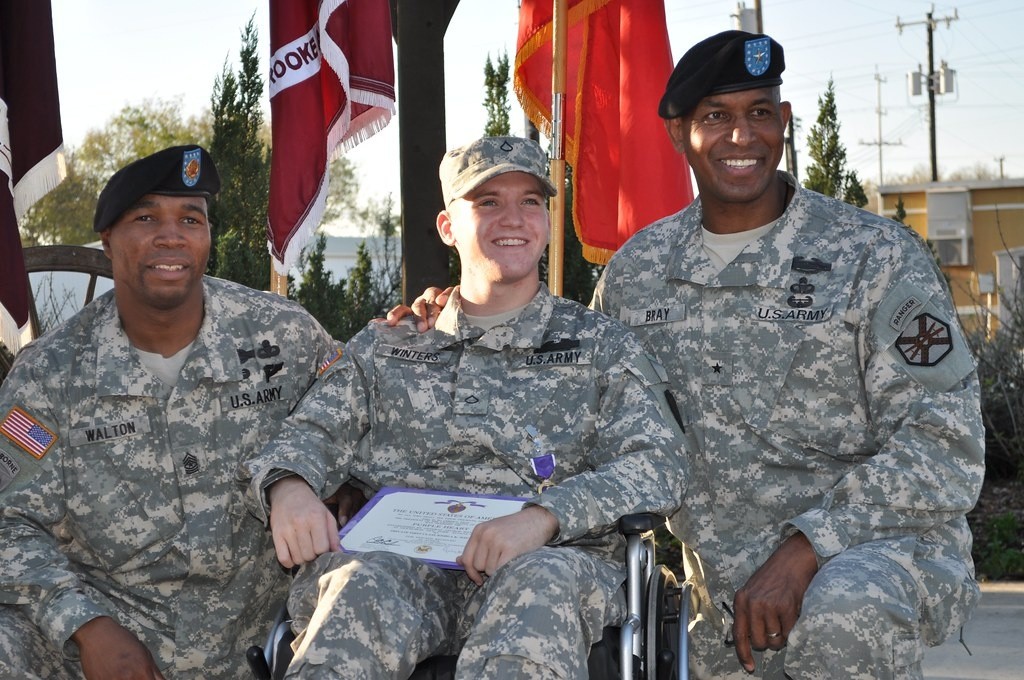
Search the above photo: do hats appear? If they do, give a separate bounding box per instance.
[438,136,557,209]
[657,30,786,119]
[93,144,221,232]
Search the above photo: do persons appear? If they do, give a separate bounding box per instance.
[383,30,985,680]
[234,137,692,680]
[0,145,371,680]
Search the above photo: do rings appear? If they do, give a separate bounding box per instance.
[768,633,779,639]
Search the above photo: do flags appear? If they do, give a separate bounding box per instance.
[267,0,396,278]
[0,0,68,357]
[515,0,695,266]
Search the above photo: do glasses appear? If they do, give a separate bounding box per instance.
[721,601,752,645]
